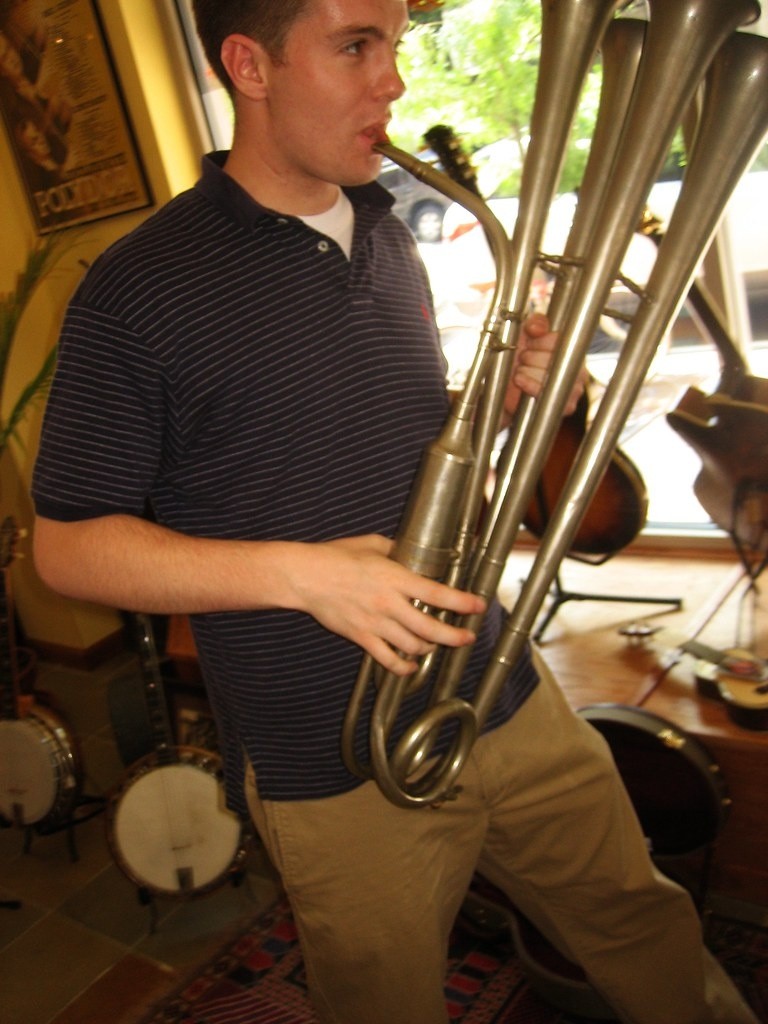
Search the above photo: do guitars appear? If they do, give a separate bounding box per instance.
[417,122,649,561]
[582,184,768,553]
[616,617,768,731]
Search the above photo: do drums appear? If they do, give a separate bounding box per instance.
[102,742,247,903]
[1,706,82,836]
[501,703,737,997]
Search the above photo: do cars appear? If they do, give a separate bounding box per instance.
[442,106,768,352]
[373,120,488,242]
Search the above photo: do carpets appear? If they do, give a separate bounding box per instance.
[124,874,768,1024]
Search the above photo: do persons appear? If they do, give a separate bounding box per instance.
[26,0,767,1024]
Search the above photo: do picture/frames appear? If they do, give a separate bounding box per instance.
[0,0,153,237]
